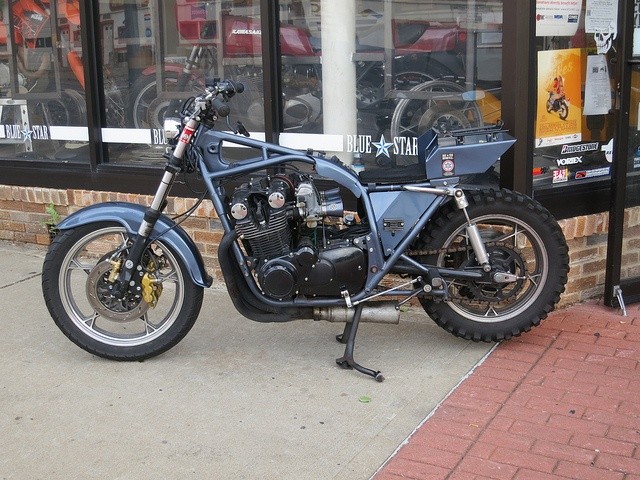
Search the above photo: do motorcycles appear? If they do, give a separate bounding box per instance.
[545,91,569,121]
[129,1,470,151]
[39,79,570,384]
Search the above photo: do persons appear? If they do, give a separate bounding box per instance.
[547,78,560,112]
[16,39,51,94]
[550,74,565,111]
